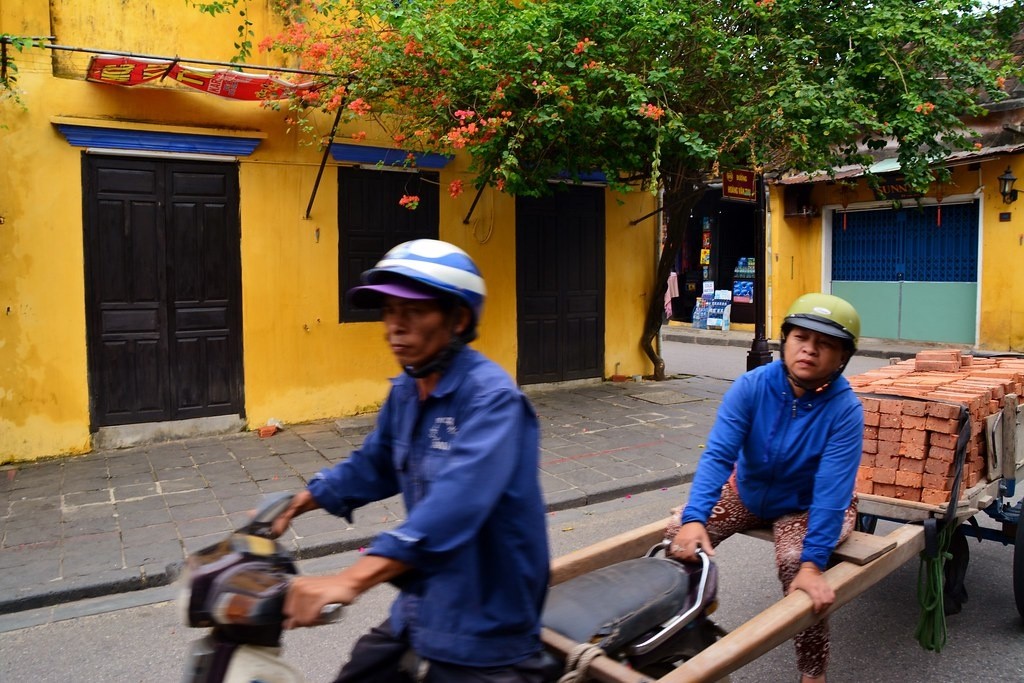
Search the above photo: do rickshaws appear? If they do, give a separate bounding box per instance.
[538,357,1024,683]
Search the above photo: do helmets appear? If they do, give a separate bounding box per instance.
[360,238,488,327]
[780,292,860,350]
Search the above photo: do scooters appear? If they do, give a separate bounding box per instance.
[167,491,730,683]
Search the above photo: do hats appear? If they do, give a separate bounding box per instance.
[345,275,465,308]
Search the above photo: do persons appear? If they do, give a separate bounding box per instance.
[664,293,864,683]
[248,239,551,683]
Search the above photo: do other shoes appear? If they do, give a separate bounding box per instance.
[800,673,827,683]
[703,597,718,616]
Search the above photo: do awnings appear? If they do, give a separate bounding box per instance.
[773,142,1024,184]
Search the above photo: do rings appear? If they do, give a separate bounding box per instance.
[671,545,683,552]
